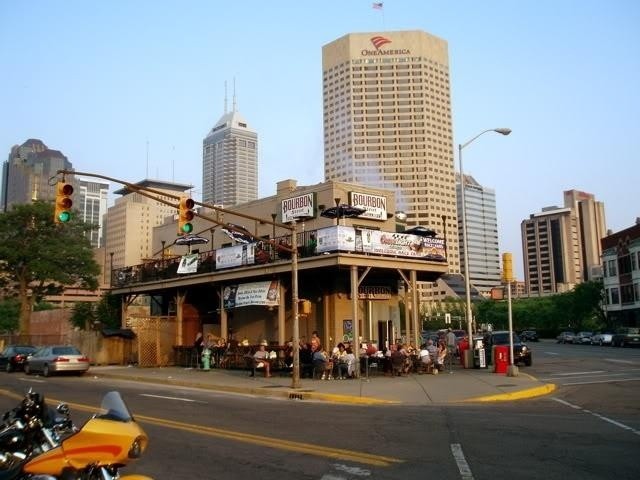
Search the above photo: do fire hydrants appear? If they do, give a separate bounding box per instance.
[202,349,211,371]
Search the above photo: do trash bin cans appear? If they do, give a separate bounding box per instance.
[459,338,470,363]
[493,345,510,373]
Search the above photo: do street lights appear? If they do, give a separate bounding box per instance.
[458,128,511,351]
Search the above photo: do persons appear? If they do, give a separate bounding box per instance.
[256,232,317,265]
[193,327,493,381]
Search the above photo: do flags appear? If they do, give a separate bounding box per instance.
[372,2,383,11]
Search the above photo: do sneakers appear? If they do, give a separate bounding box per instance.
[321,374,334,381]
[336,375,347,380]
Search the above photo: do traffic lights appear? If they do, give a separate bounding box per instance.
[178,198,194,234]
[54,180,74,222]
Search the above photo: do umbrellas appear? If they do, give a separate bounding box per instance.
[171,232,210,254]
[320,201,368,225]
[221,223,254,245]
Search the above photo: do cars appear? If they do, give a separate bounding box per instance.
[1,346,37,372]
[438,330,538,366]
[557,328,640,347]
[24,345,90,376]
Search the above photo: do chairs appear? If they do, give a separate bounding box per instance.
[172,343,446,377]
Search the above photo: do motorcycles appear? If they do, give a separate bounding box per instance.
[0,389,148,480]
